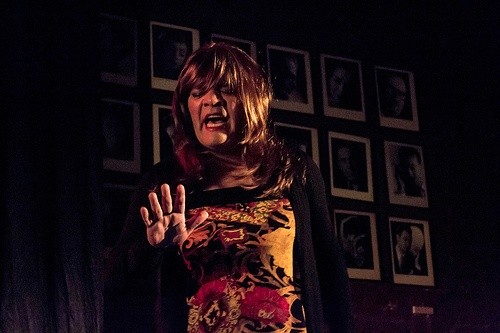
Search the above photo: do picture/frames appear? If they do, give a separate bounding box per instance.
[333,208,384,282]
[388,216,436,288]
[374,65,420,134]
[100,182,140,245]
[266,43,315,116]
[96,98,142,175]
[273,121,321,171]
[148,19,201,91]
[320,53,367,123]
[327,130,375,204]
[93,10,139,88]
[383,139,429,210]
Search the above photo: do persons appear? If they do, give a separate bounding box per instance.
[154,28,189,80]
[104,42,357,333]
[332,143,363,191]
[393,224,421,276]
[393,146,427,197]
[336,215,374,269]
[269,52,303,102]
[380,75,407,118]
[325,57,360,111]
